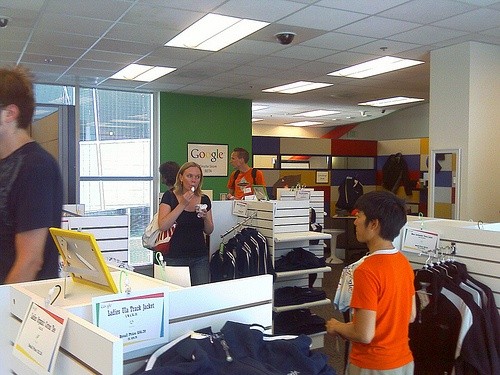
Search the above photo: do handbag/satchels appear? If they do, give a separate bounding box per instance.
[142,190,176,256]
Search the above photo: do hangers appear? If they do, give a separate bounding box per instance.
[412,246,458,296]
[217,215,256,254]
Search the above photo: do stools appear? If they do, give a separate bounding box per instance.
[324,229,345,264]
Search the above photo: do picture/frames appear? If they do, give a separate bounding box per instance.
[252,185,269,201]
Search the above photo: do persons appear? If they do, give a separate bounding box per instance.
[0,65,65,286]
[224,148,267,202]
[324,189,420,375]
[160,161,215,287]
[158,161,181,205]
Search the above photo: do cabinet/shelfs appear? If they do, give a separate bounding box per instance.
[0,264,273,375]
[58,204,130,278]
[277,188,324,287]
[210,200,332,350]
[392,215,500,317]
